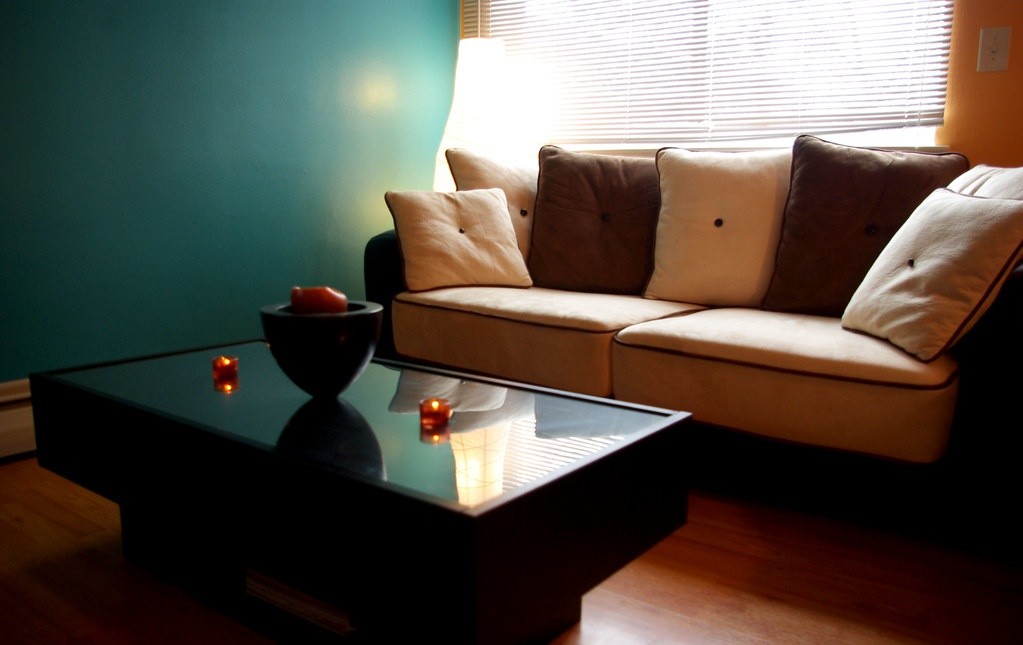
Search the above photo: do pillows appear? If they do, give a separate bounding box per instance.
[759,134,969,320]
[530,146,660,296]
[642,147,791,311]
[842,187,1023,364]
[384,188,533,293]
[446,150,534,272]
[945,163,1023,200]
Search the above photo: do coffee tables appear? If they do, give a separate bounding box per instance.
[30,337,694,644]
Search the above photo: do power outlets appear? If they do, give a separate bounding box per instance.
[977,25,1012,72]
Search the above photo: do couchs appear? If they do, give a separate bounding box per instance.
[364,228,1023,553]
